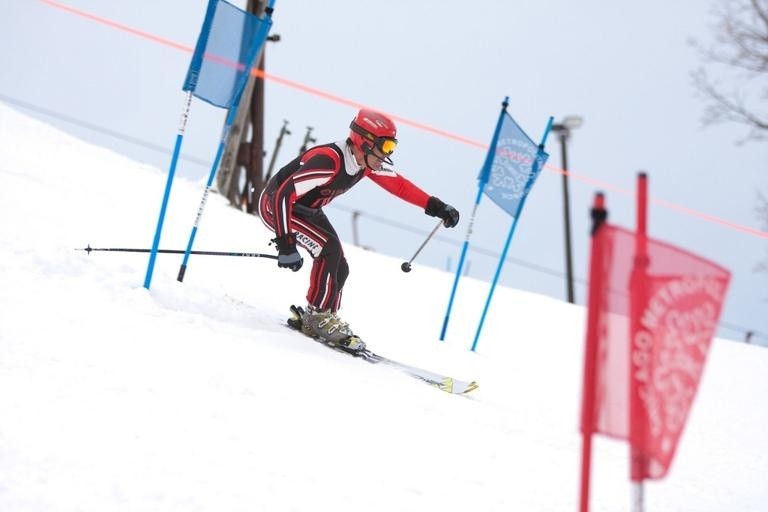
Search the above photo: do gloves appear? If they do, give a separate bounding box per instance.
[424,196,459,227]
[272,232,302,272]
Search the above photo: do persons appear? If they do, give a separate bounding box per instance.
[260,105,461,350]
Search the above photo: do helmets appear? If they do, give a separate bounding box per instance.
[349,109,396,156]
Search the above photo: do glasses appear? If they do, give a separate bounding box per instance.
[349,122,397,156]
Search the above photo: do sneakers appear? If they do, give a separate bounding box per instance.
[303,307,351,341]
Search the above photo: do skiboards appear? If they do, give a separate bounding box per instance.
[278,304,477,398]
[217,0,264,215]
